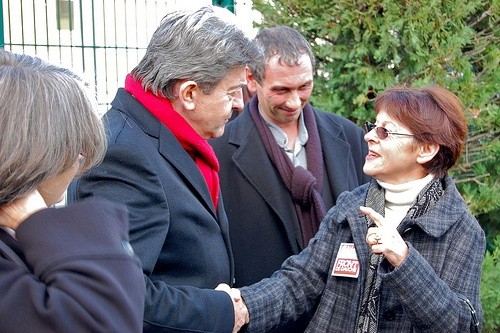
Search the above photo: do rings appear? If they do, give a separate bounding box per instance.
[374,233,382,244]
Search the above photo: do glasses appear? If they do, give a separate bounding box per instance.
[361,121,415,140]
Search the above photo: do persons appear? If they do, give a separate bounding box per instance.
[0,49,147,333]
[66,7,261,333]
[203,23,371,333]
[231,83,486,333]
